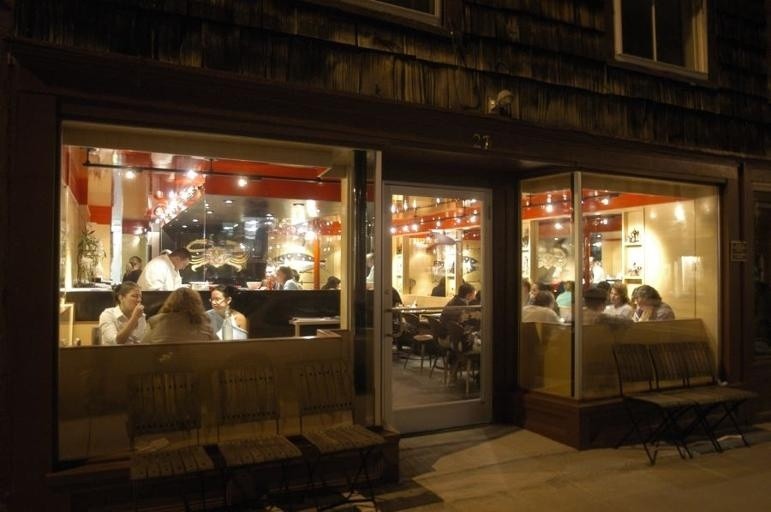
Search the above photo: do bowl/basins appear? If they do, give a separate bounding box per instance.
[246,282,262,290]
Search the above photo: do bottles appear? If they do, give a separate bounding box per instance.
[221,309,233,341]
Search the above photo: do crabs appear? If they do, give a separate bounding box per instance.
[186,239,251,272]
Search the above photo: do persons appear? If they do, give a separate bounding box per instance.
[320,276,343,291]
[257,264,282,290]
[137,248,193,291]
[160,249,173,257]
[99,279,151,348]
[141,287,220,343]
[275,266,301,290]
[279,269,300,290]
[247,308,290,339]
[433,280,676,388]
[366,253,375,283]
[121,256,144,284]
[391,285,404,323]
[205,283,248,341]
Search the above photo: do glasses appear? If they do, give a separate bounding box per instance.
[209,297,227,306]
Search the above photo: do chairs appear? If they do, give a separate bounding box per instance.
[611,340,760,465]
[213,363,303,510]
[402,312,481,393]
[293,358,387,512]
[126,368,213,511]
